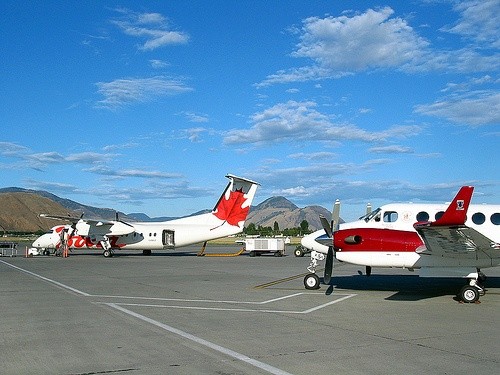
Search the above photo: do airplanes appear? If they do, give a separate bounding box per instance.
[295,181,500,303]
[31,171,261,258]
